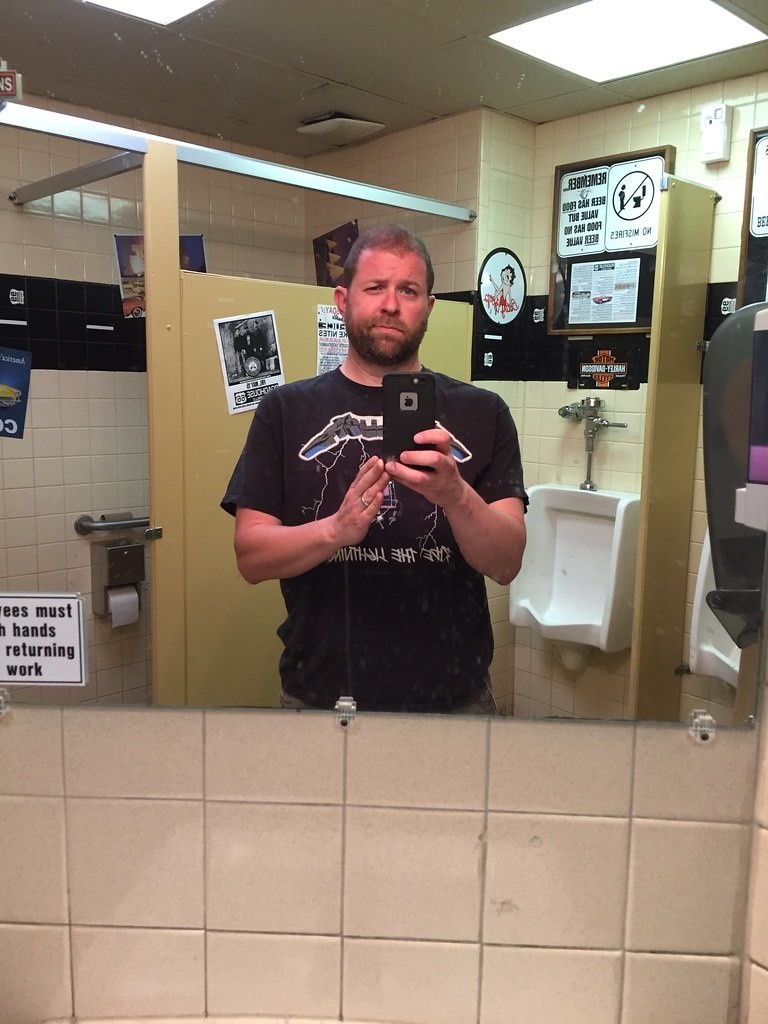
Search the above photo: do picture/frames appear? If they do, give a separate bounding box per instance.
[546,145,677,336]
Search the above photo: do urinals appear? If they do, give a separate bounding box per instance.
[508,483,642,654]
[688,523,741,688]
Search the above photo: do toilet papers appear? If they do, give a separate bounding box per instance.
[107,584,140,629]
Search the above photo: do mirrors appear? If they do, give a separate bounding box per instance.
[1,0,768,732]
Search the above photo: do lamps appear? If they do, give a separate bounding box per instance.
[299,114,387,143]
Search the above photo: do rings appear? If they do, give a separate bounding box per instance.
[361,496,370,506]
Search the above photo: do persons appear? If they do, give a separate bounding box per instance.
[218,227,531,714]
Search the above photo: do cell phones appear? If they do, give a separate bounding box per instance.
[382,373,437,469]
